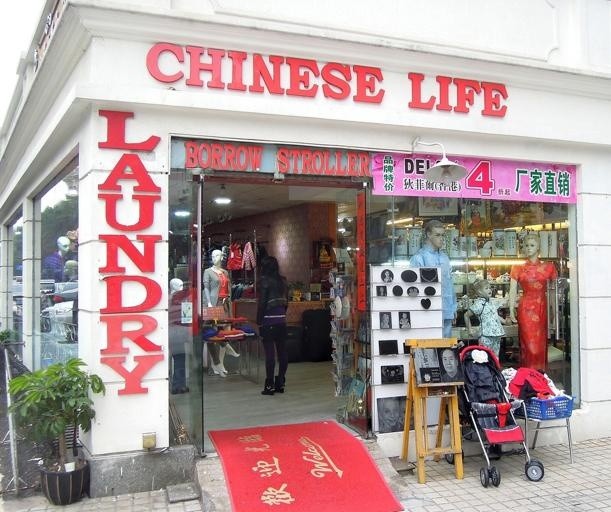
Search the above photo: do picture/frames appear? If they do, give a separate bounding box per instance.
[417,195,460,218]
[465,198,487,232]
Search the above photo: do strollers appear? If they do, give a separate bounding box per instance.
[447,341,545,488]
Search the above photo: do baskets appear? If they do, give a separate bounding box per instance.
[514,392,574,420]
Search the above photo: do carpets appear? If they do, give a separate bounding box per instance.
[207,419,405,512]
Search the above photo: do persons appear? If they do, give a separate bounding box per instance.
[442,350,462,382]
[378,397,404,433]
[169,250,240,396]
[463,280,508,359]
[411,221,458,337]
[62,257,78,306]
[42,236,73,305]
[256,255,291,395]
[399,400,413,430]
[509,234,560,372]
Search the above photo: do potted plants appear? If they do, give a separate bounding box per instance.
[5,357,106,507]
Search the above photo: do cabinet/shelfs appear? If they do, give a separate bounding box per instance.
[453,281,519,340]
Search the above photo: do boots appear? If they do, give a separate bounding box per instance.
[261,378,275,395]
[275,376,285,393]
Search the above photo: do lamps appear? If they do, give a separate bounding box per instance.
[413,136,467,186]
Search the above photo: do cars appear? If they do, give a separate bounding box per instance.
[11,276,78,341]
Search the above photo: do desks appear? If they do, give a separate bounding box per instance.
[203,331,261,384]
